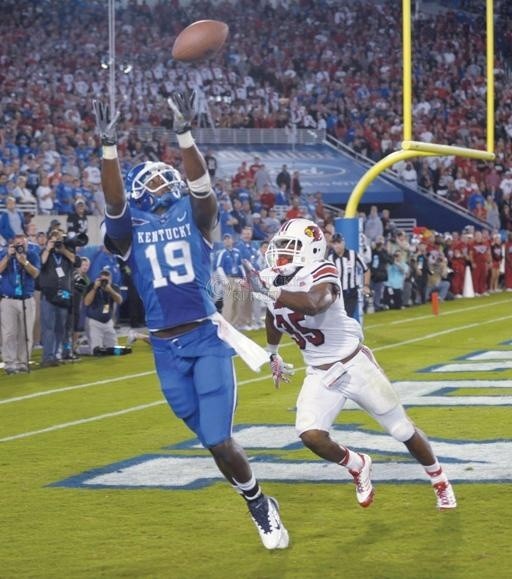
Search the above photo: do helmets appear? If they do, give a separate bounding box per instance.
[265,218,326,276]
[125,161,186,212]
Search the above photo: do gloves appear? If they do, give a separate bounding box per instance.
[167,89,196,134]
[270,353,295,389]
[234,258,271,296]
[92,99,121,145]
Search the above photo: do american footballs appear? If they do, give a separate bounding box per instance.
[171,20,229,61]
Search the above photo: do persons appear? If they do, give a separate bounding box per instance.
[266,217,457,516]
[0,0,512,370]
[91,98,290,551]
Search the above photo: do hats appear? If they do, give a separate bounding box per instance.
[329,233,344,242]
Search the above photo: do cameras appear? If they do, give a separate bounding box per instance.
[12,246,23,254]
[101,279,109,287]
[376,242,382,248]
[63,233,89,252]
[393,253,399,257]
[52,240,62,248]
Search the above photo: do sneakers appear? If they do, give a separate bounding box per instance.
[247,496,290,550]
[349,453,374,508]
[432,480,457,509]
